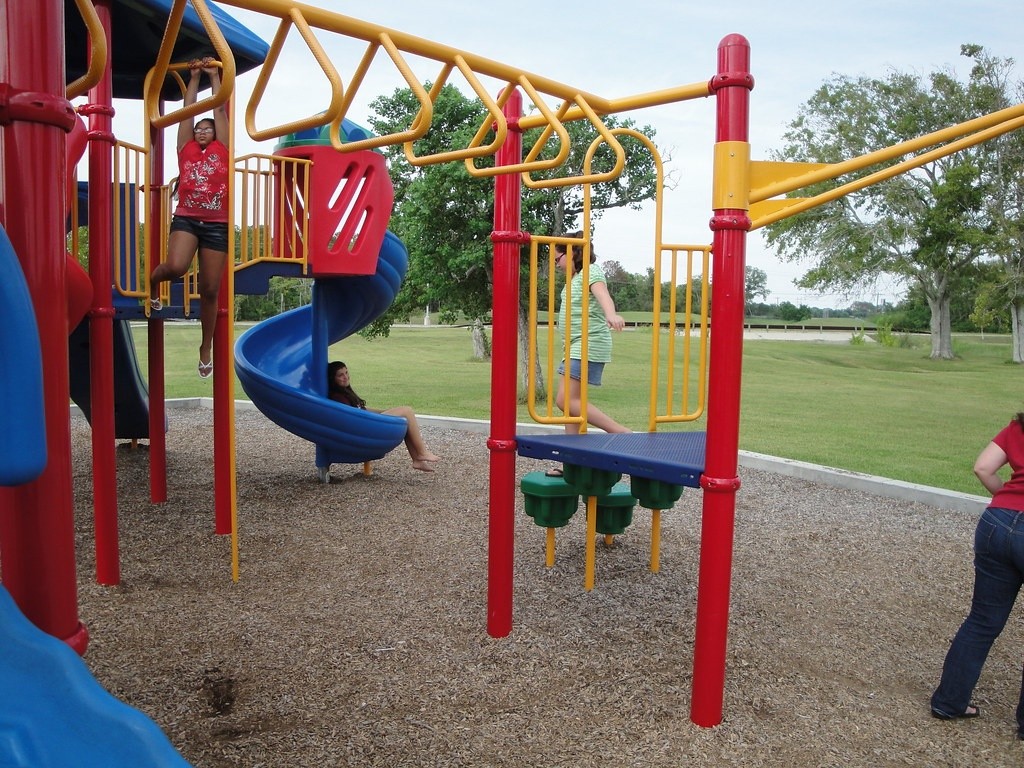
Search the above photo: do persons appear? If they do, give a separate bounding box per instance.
[546,230,636,478]
[150,57,230,377]
[930,413,1024,742]
[327,361,440,472]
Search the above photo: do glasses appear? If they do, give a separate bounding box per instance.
[193,127,214,133]
[555,252,566,262]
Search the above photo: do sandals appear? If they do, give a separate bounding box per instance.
[932,704,980,718]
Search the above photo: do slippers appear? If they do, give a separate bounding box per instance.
[150,297,162,311]
[544,468,564,476]
[198,360,213,378]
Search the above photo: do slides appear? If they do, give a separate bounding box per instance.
[235,217,406,467]
[69,311,169,441]
[2,230,185,768]
